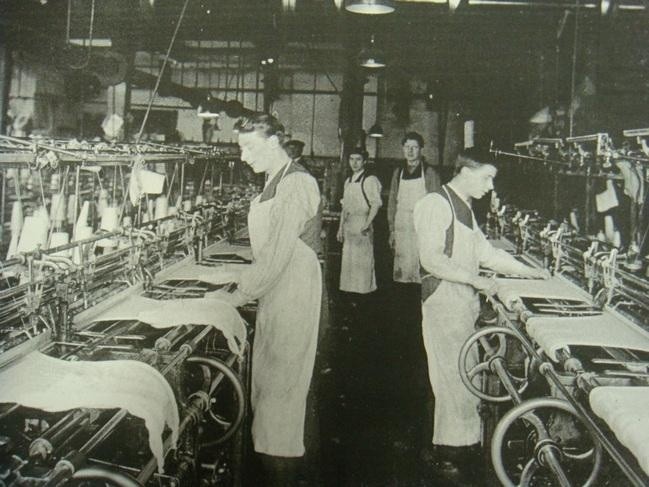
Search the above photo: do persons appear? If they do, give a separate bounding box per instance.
[285,139,306,166]
[335,149,383,322]
[388,132,438,311]
[205,110,330,487]
[416,148,547,486]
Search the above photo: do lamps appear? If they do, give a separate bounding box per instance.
[367,117,386,139]
[344,0,400,16]
[355,22,388,69]
[196,38,220,121]
